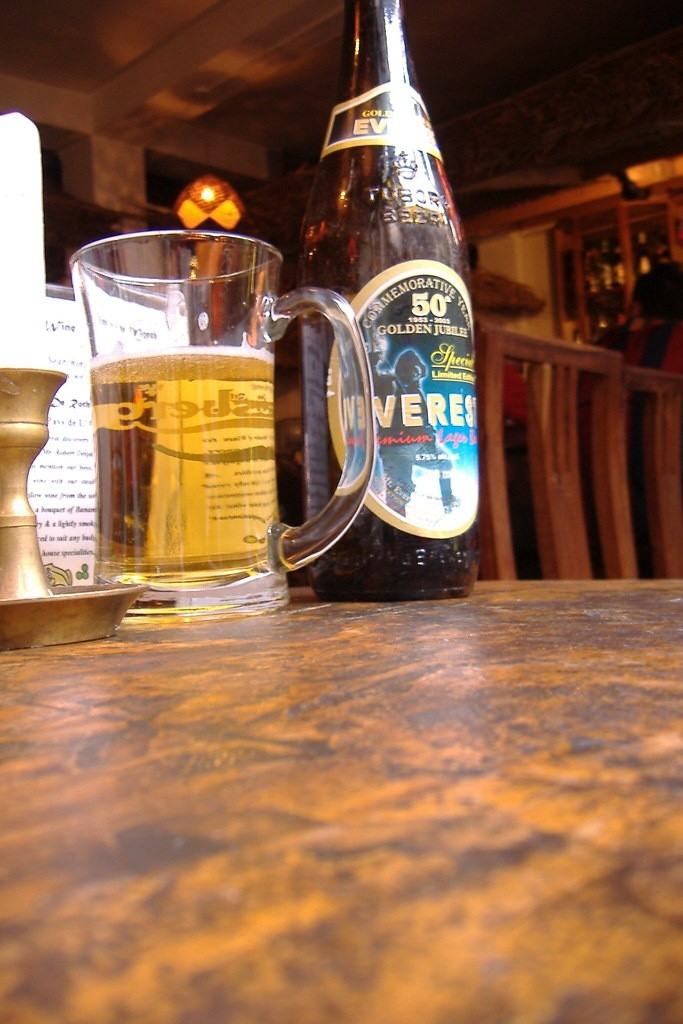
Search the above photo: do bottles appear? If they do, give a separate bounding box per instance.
[581,229,670,344]
[300,0,479,601]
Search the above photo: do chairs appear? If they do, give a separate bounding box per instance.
[469,324,682,582]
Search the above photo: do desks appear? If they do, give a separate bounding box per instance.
[0,580,683,1024]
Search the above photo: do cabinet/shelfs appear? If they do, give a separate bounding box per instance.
[548,189,683,349]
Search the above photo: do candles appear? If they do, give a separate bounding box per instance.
[0,111,49,370]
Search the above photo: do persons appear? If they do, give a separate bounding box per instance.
[275,438,307,533]
[606,252,683,371]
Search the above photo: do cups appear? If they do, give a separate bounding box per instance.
[69,229,375,620]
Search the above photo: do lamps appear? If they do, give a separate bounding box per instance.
[174,176,248,232]
